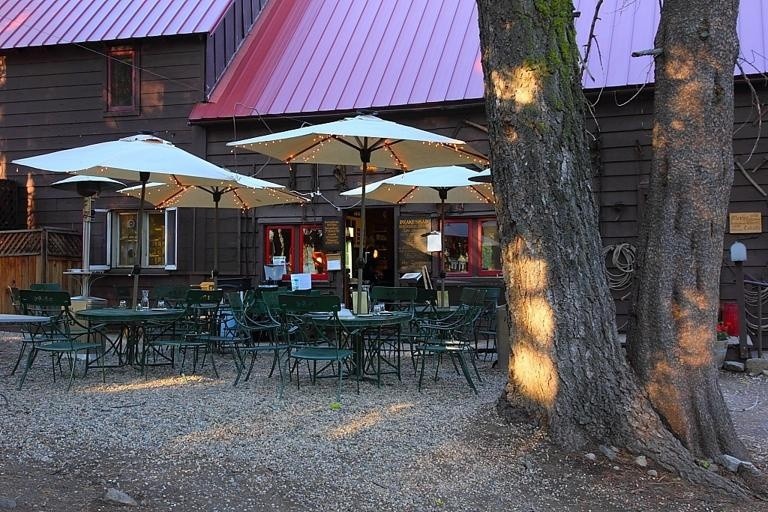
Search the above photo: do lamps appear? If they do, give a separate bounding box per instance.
[427,230,442,286]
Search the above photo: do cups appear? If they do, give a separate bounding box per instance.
[120,300,127,308]
[158,300,165,309]
[140,290,149,308]
[378,302,384,311]
[374,305,380,314]
[136,306,143,311]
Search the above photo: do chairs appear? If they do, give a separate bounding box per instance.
[226,292,276,361]
[30,283,100,369]
[408,287,481,375]
[140,289,224,377]
[261,289,313,378]
[277,293,360,403]
[416,289,488,395]
[15,288,106,393]
[364,286,417,373]
[6,284,76,377]
